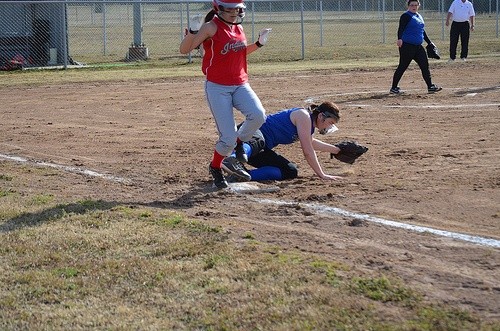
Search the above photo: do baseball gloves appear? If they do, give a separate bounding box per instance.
[330,140,368,164]
[425,43,440,60]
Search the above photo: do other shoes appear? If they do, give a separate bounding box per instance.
[460,58,466,62]
[448,58,456,62]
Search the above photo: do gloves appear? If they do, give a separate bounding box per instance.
[190,11,203,31]
[258,28,273,45]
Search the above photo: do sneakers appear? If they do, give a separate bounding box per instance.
[389,87,400,94]
[208,162,227,188]
[427,84,442,93]
[222,157,252,181]
[234,143,248,163]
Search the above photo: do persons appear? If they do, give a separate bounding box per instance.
[221,99,369,180]
[388,0,442,95]
[445,0,475,62]
[180,0,272,188]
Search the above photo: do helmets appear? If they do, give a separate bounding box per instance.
[212,0,246,18]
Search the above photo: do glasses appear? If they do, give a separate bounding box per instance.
[409,4,417,6]
